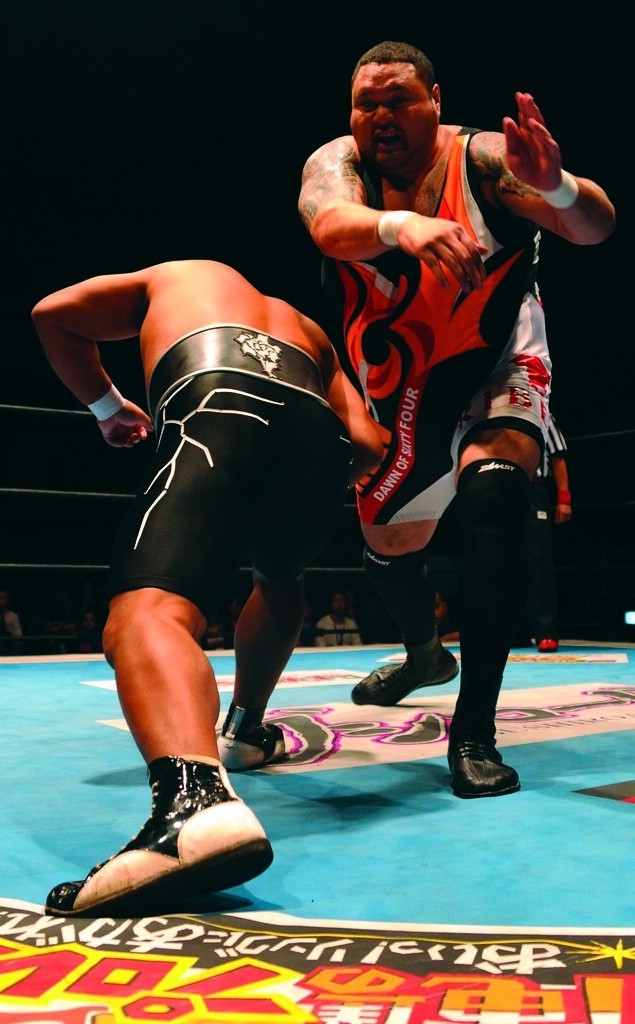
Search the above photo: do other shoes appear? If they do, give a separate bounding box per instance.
[539,639,558,651]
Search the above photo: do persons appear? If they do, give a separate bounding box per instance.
[30,260,384,917]
[314,591,362,646]
[432,590,460,642]
[296,40,615,797]
[0,590,244,654]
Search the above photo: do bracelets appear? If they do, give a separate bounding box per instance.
[536,168,579,209]
[87,384,125,422]
[378,210,417,247]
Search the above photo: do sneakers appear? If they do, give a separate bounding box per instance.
[45,755,273,916]
[448,722,519,797]
[352,642,459,706]
[218,702,286,772]
